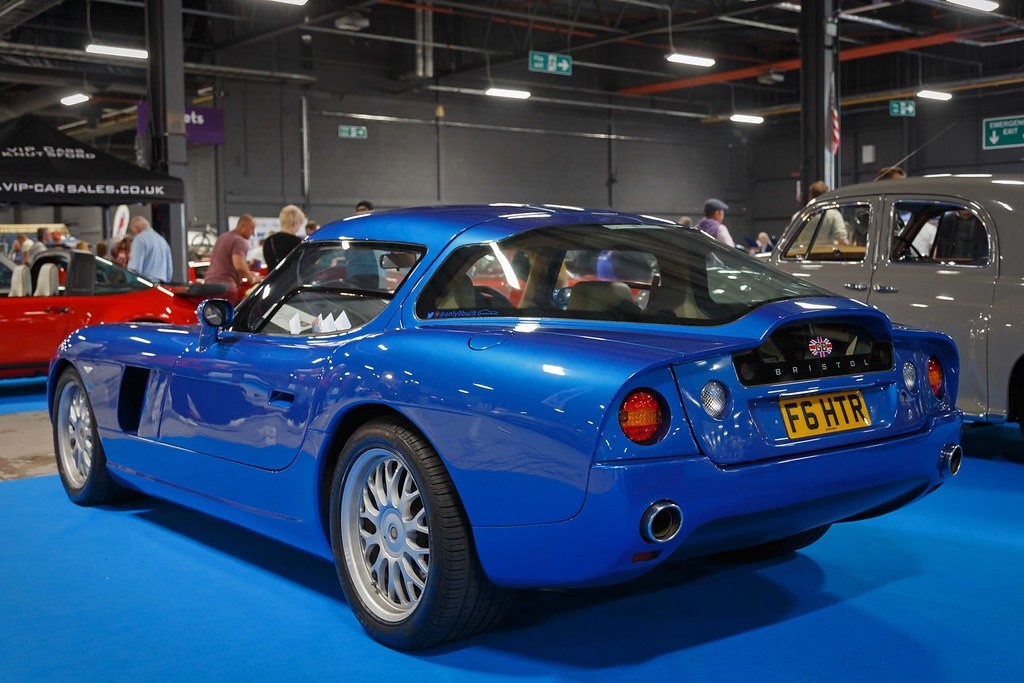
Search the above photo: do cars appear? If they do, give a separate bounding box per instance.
[46,204,964,649]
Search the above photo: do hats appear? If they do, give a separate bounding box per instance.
[704,199,730,214]
[357,201,373,211]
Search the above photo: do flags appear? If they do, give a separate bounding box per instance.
[830,71,841,156]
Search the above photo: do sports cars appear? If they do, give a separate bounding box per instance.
[0,246,655,379]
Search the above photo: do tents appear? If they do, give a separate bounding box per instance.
[0,113,189,285]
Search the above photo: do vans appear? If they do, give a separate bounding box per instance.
[754,176,1024,424]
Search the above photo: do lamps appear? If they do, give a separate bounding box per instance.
[85,44,148,59]
[484,87,531,99]
[60,93,89,106]
[917,90,952,102]
[667,53,716,68]
[730,114,764,125]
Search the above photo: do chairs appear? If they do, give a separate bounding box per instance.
[434,271,476,311]
[33,263,58,299]
[8,264,31,299]
[569,280,633,316]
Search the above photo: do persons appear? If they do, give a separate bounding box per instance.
[693,198,735,248]
[573,250,653,284]
[250,239,266,264]
[203,213,266,295]
[909,217,939,258]
[261,204,319,297]
[127,216,174,284]
[77,234,134,287]
[678,216,692,226]
[306,222,316,235]
[757,232,774,253]
[855,166,912,248]
[10,227,72,268]
[343,200,388,290]
[930,208,988,265]
[790,180,851,247]
